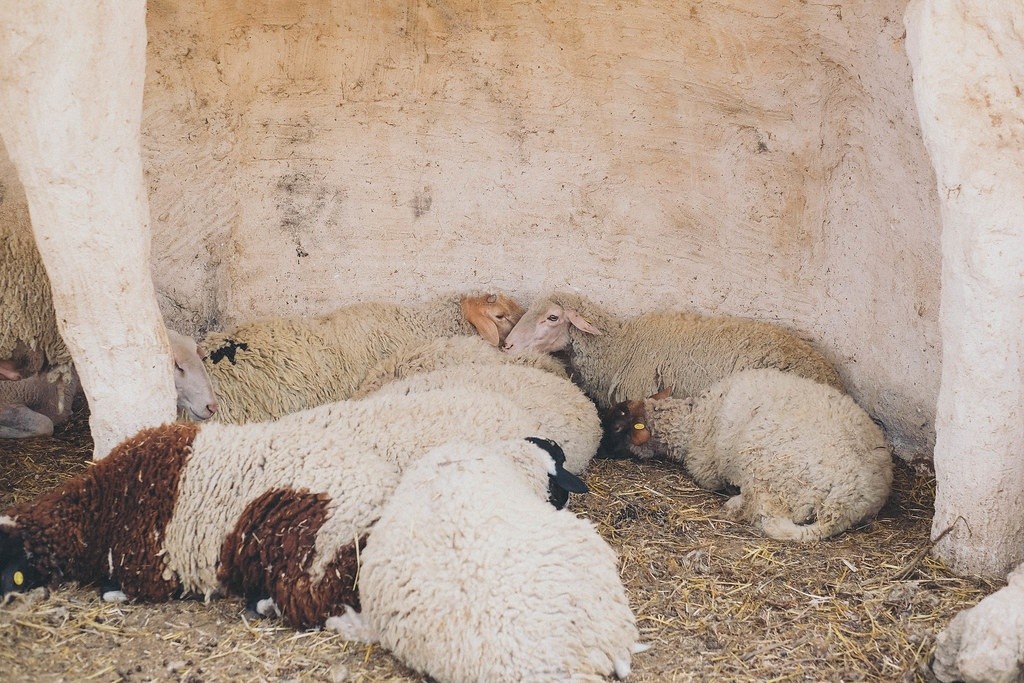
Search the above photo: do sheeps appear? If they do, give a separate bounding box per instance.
[0,216,217,436]
[184,294,566,425]
[0,365,640,683]
[501,294,893,541]
